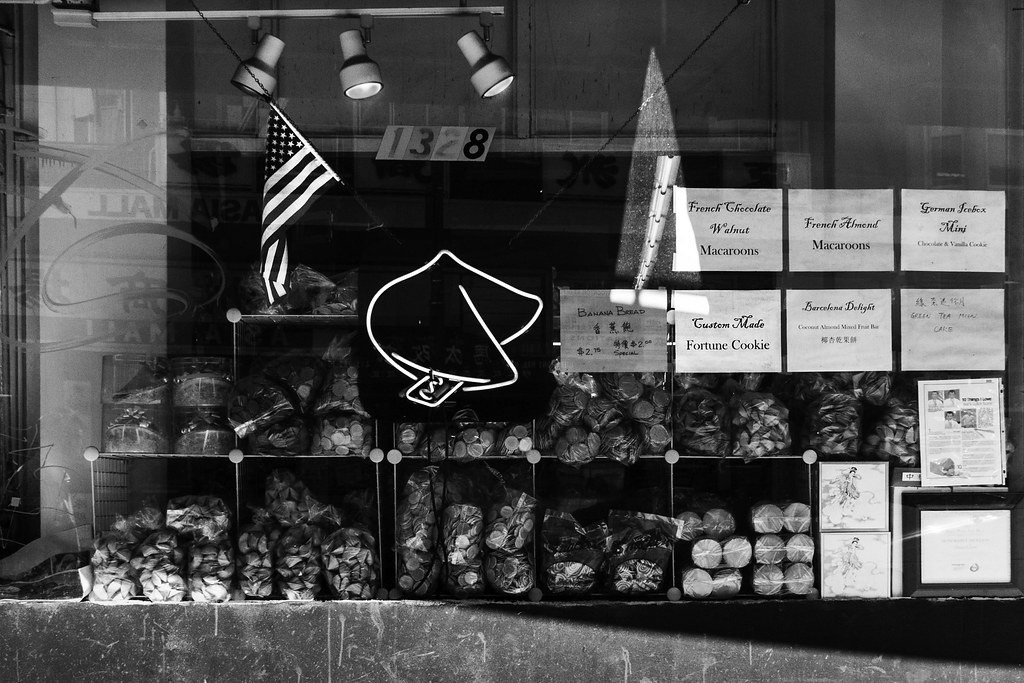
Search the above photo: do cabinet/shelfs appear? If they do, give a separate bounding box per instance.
[86,305,820,605]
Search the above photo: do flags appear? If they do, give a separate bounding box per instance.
[259,103,339,307]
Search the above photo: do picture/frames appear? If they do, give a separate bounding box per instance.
[818,459,889,532]
[820,533,891,598]
[904,493,1024,597]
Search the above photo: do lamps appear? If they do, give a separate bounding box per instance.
[339,14,383,100]
[231,13,284,102]
[633,153,683,290]
[455,11,516,99]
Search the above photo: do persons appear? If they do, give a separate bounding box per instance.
[945,411,961,428]
[928,391,943,407]
[945,389,960,407]
